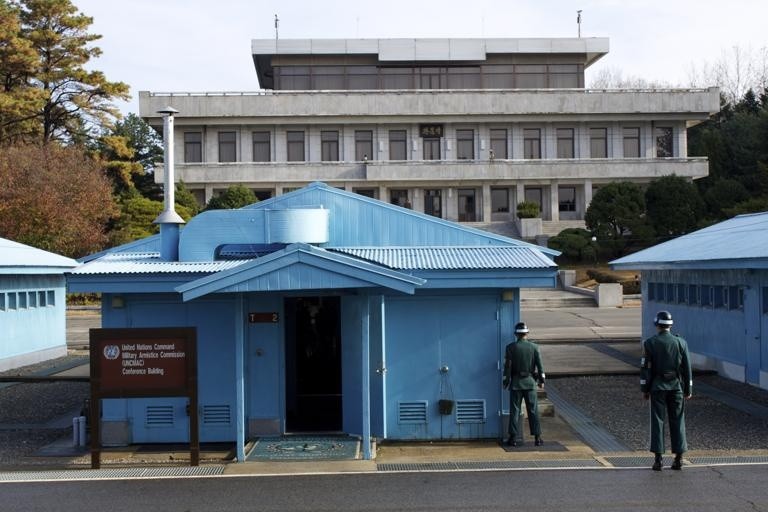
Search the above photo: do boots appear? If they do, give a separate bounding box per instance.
[534,434,545,446]
[670,455,684,471]
[505,433,518,447]
[651,453,664,471]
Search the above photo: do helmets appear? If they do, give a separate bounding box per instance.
[513,322,530,335]
[653,310,673,328]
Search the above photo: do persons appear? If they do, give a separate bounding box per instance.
[638,311,694,470]
[501,322,546,446]
